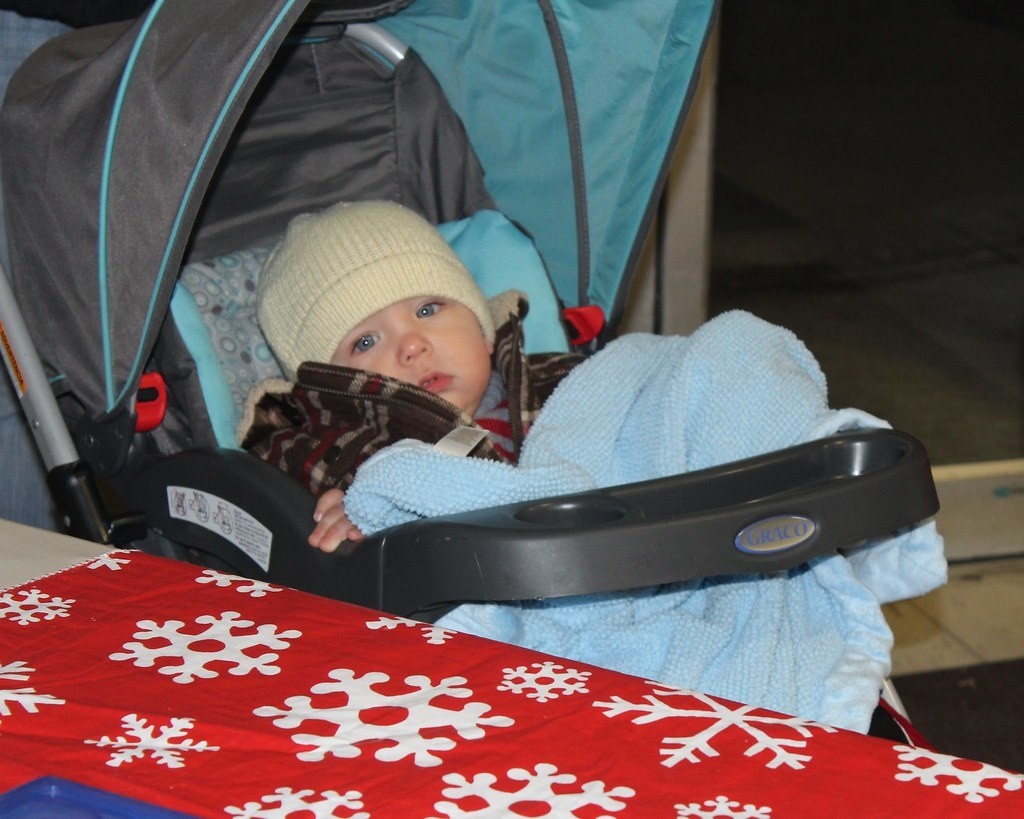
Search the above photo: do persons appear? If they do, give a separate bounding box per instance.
[239,198,946,735]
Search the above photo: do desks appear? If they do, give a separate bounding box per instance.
[0,518,1024,819]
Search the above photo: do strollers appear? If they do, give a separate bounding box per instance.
[0,0,941,748]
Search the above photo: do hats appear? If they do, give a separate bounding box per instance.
[256,200,495,382]
